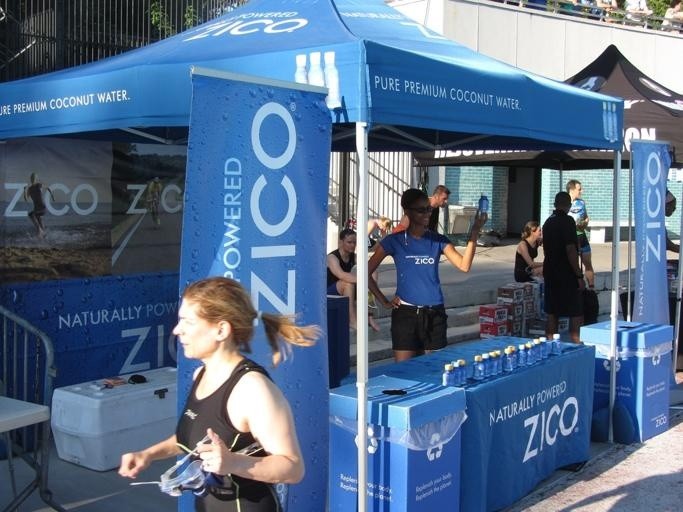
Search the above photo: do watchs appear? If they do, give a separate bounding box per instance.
[576,273,584,279]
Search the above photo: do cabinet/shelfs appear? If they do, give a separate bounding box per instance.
[667,293,683,354]
[343,335,595,511]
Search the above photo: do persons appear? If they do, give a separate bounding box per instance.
[664,188,679,253]
[390,184,450,235]
[145,177,165,229]
[367,188,488,364]
[366,216,390,237]
[514,220,544,282]
[23,173,55,239]
[565,180,595,290]
[499,0,683,31]
[326,229,384,333]
[542,192,586,343]
[117,277,320,511]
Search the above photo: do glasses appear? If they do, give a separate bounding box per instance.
[158,434,212,498]
[405,206,433,213]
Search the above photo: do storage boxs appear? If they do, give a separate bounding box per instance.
[51,366,178,472]
[478,272,585,337]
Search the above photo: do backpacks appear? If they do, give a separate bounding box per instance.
[582,289,600,321]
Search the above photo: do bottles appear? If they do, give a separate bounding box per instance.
[441,333,561,387]
[478,192,489,213]
[291,50,342,113]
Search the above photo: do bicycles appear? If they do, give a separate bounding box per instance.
[148,192,162,229]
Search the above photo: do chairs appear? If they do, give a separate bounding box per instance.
[618,290,634,323]
[0,305,71,512]
